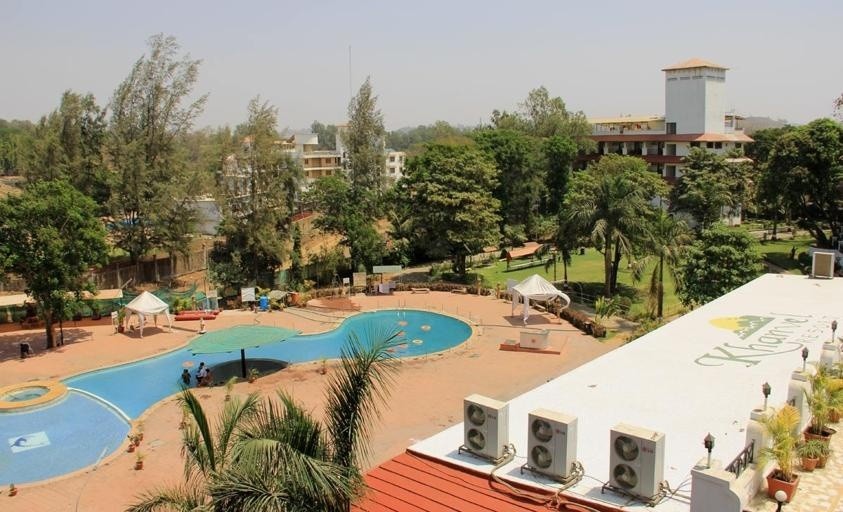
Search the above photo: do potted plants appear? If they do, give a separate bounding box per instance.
[754,362,842,504]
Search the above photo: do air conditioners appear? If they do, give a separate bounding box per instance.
[609,421,667,499]
[527,408,577,477]
[461,393,511,459]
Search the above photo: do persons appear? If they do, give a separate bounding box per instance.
[181,369,192,387]
[197,362,214,387]
